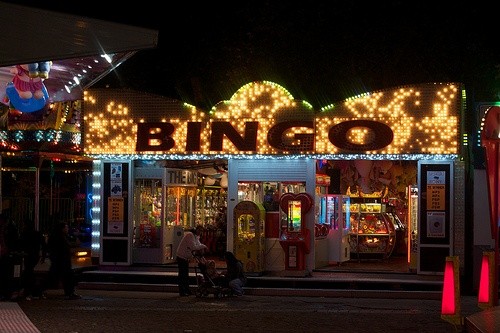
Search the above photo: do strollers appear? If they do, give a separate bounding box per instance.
[188,247,226,298]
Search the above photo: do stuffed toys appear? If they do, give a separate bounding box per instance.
[334,160,418,195]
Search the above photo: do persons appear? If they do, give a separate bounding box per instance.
[206,251,247,297]
[177,226,207,296]
[0,219,83,300]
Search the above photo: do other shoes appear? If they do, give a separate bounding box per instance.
[64,292,81,298]
[39,293,47,299]
[232,291,244,296]
[180,290,193,296]
